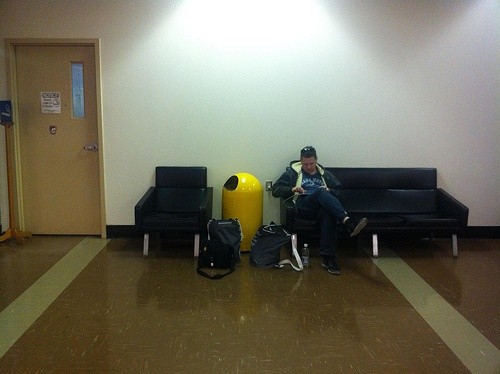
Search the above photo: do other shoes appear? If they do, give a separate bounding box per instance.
[321,257,342,275]
[344,217,368,237]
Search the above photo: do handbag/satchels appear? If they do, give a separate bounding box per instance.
[249,222,304,272]
[197,218,241,279]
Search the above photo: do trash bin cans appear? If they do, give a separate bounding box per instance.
[222,173,263,252]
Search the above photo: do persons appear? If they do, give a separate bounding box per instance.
[272,146,368,274]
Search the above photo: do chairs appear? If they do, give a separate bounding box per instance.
[134,165,213,257]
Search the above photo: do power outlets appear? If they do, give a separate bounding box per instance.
[266,180,272,192]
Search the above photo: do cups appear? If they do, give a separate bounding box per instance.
[265,180,272,190]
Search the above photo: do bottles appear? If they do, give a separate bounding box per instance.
[301,244,310,267]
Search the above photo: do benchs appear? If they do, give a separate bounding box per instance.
[280,167,469,258]
